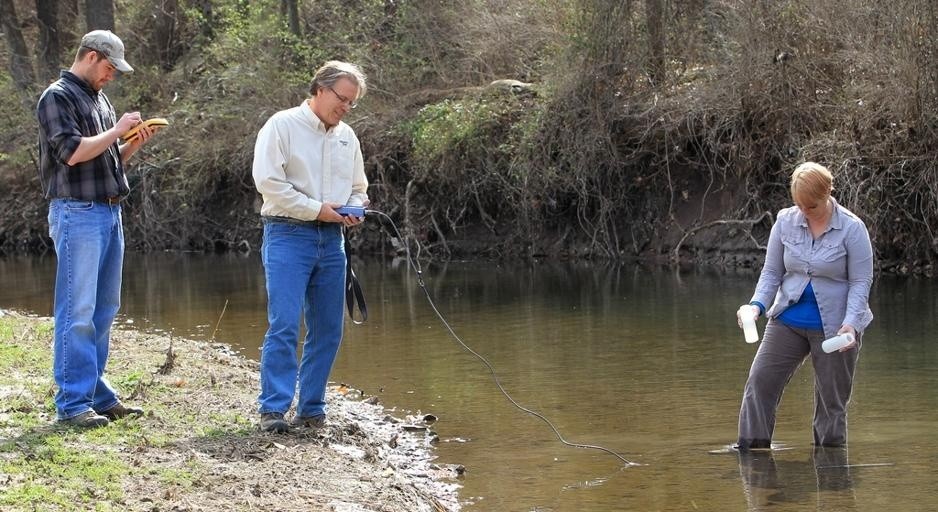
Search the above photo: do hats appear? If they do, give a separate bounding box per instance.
[80,29,134,73]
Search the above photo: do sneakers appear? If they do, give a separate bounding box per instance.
[101,403,143,421]
[291,415,331,429]
[58,411,108,429]
[260,412,288,432]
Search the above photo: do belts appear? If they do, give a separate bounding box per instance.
[102,196,120,204]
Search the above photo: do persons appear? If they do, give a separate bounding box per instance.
[34,28,160,432]
[250,57,371,432]
[736,160,873,449]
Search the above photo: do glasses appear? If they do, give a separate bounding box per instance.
[328,85,358,109]
